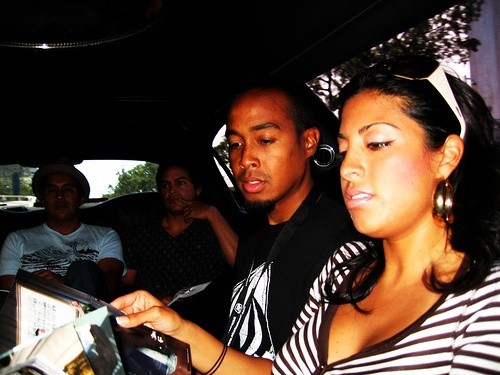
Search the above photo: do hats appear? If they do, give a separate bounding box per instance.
[32,163,90,207]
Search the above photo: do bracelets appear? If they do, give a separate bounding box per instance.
[209,346,228,375]
[200,344,226,375]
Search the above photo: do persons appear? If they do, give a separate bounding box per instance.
[0,162,129,305]
[108,55,500,375]
[118,161,239,342]
[218,69,368,362]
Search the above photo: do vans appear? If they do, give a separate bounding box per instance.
[0,195,37,211]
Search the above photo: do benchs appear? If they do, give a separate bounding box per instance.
[0,192,212,253]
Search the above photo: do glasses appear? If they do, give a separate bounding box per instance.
[391,58,466,140]
